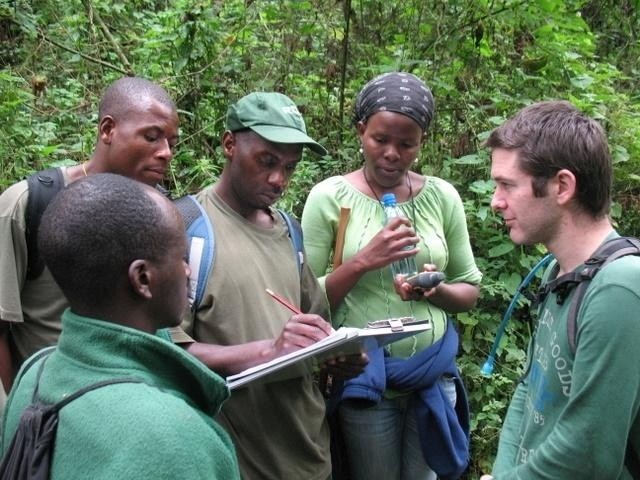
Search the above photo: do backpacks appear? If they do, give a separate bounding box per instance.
[0,353,148,480]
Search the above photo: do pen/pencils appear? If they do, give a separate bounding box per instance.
[264,288,304,316]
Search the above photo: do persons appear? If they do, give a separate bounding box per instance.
[0,73,178,400]
[299,68,482,480]
[171,91,370,480]
[1,173,244,480]
[483,101,640,480]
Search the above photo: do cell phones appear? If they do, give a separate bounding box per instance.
[406,272,445,290]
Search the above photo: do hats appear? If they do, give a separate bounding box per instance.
[225,92,327,156]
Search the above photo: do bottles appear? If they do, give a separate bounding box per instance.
[380,194,417,282]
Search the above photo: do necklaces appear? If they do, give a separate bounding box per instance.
[81,161,90,177]
[363,166,418,251]
